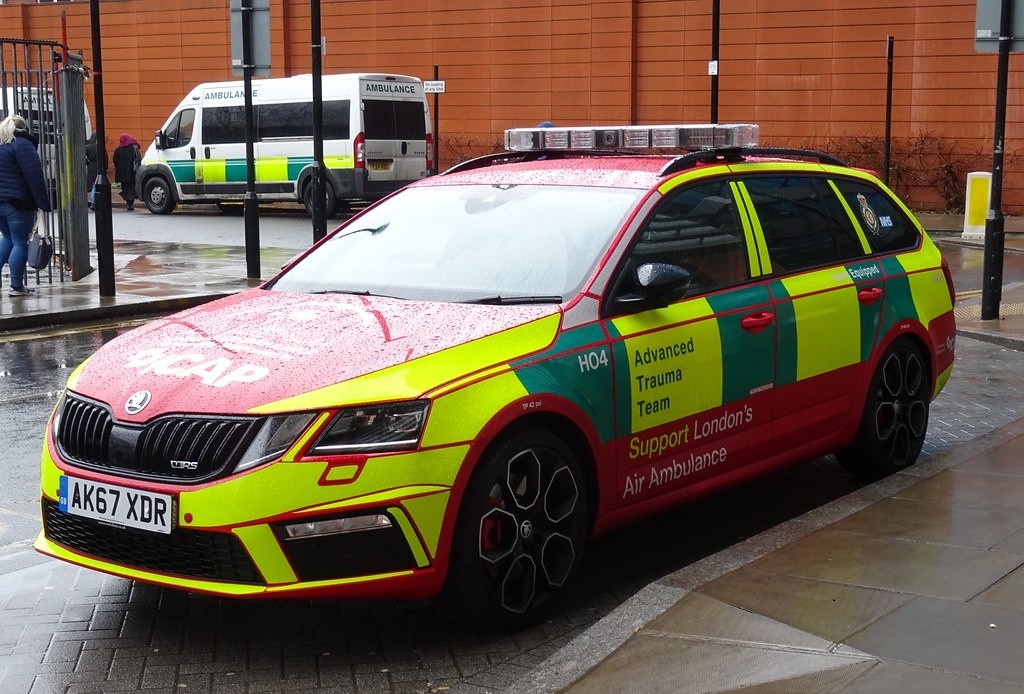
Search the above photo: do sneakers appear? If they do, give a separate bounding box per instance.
[8,285,34,296]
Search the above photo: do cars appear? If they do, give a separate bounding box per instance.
[34,129,957,636]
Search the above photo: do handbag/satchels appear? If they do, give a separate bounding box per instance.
[133,144,142,172]
[26,210,54,270]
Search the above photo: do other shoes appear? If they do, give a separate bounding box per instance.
[126,204,134,211]
[90,203,95,211]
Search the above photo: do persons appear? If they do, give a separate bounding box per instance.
[113,133,142,210]
[86,133,109,209]
[0,114,51,297]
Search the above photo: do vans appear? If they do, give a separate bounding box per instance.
[136,73,432,218]
[0,87,92,202]
